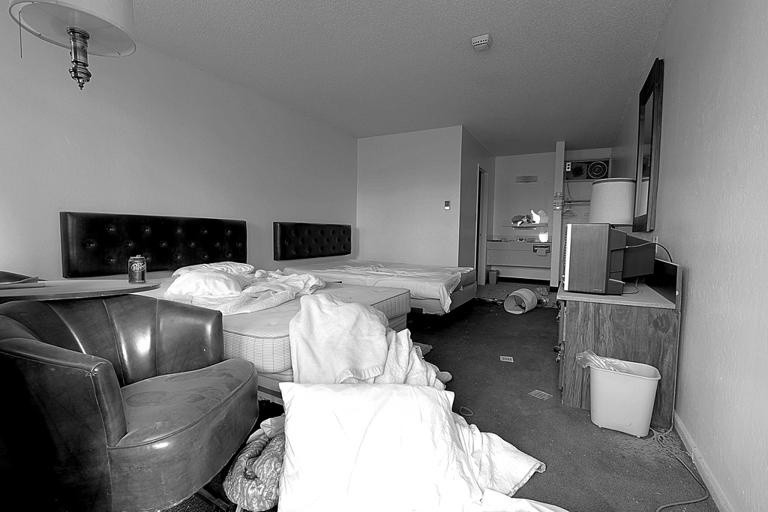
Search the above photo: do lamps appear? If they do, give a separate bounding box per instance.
[633,178,650,217]
[9,1,138,89]
[589,177,636,231]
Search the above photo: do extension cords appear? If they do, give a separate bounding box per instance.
[566,161,571,172]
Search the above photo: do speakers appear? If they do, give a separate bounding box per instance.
[586,160,609,179]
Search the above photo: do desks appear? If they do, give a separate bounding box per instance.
[2,279,162,303]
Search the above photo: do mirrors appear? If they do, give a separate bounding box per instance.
[631,59,664,234]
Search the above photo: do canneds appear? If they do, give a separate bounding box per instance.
[128,254,147,283]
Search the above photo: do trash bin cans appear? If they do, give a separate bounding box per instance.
[489,270,497,285]
[590,356,661,438]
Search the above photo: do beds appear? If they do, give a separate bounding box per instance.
[273,223,479,335]
[58,210,411,406]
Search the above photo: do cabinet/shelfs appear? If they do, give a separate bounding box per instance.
[555,276,677,435]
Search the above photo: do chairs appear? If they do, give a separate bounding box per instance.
[1,294,260,512]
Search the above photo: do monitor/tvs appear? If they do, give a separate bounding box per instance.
[562,223,656,296]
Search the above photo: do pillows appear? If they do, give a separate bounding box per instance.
[171,260,255,278]
[278,380,481,510]
[161,266,244,297]
